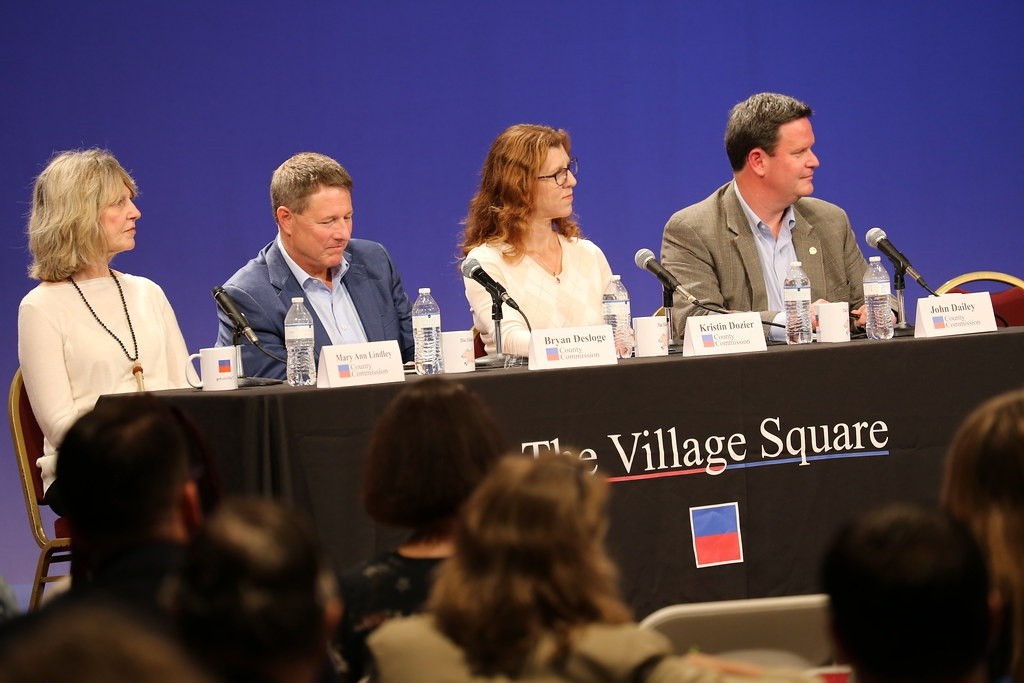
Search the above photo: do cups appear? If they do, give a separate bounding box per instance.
[440,330,478,373]
[185,347,239,392]
[815,302,851,343]
[632,316,669,357]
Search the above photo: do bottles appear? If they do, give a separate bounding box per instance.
[602,274,633,359]
[783,261,813,346]
[412,288,444,375]
[284,297,316,386]
[862,256,894,341]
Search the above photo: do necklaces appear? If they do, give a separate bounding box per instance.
[534,252,560,283]
[70,269,139,360]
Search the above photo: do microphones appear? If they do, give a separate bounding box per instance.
[865,228,927,287]
[634,248,699,305]
[211,286,259,345]
[461,258,519,310]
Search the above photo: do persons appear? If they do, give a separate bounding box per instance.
[659,91,899,346]
[18,146,200,517]
[0,377,1024,683]
[457,123,633,357]
[214,151,415,381]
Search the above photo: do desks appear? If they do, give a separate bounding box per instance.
[83,324,1023,663]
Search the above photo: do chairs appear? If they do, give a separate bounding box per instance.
[469,326,488,359]
[933,270,1024,326]
[653,306,665,316]
[7,366,72,613]
[637,594,838,667]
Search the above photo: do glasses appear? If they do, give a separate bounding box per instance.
[537,156,578,186]
